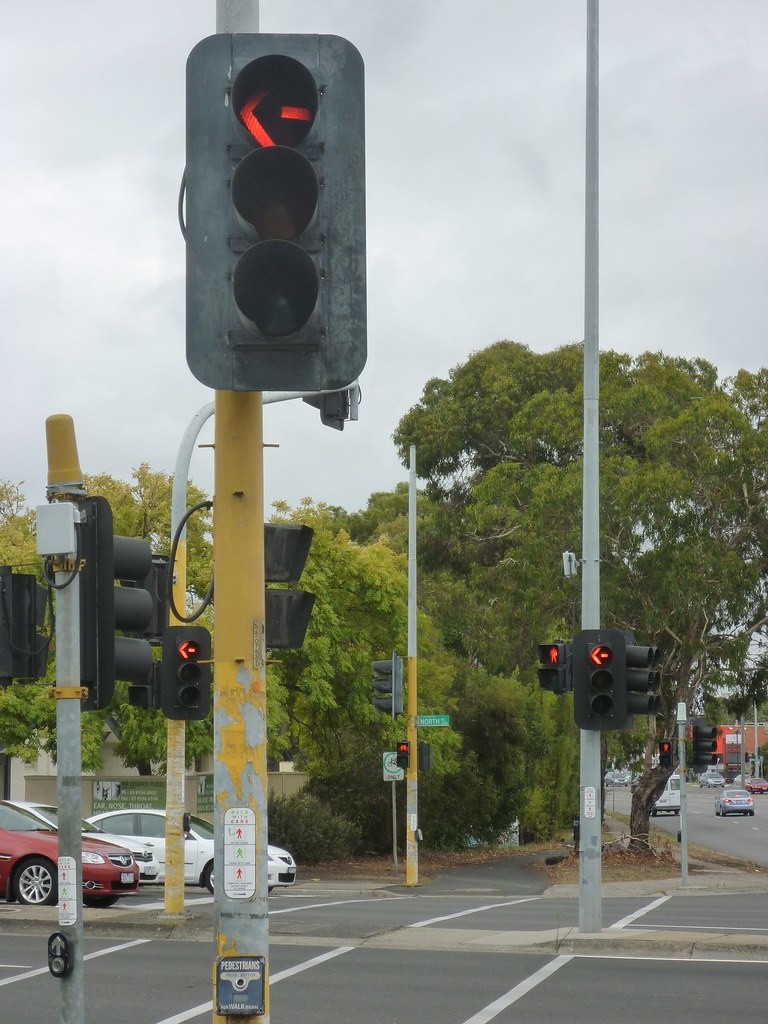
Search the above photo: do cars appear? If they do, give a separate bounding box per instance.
[605,772,628,788]
[0,802,141,907]
[89,809,297,895]
[698,772,725,788]
[733,774,768,794]
[5,800,160,886]
[715,789,754,817]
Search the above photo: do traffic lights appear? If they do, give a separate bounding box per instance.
[573,629,629,730]
[538,643,567,694]
[621,629,663,715]
[693,718,717,773]
[263,522,318,648]
[78,496,154,710]
[161,625,210,720]
[659,742,673,766]
[371,651,403,722]
[0,574,50,681]
[184,33,369,391]
[396,742,410,768]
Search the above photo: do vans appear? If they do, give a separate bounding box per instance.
[648,775,680,816]
